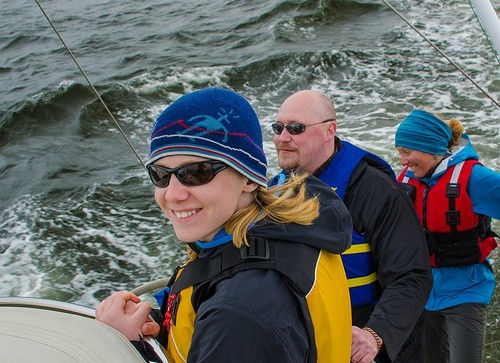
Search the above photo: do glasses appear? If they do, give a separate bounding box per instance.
[272,119,335,135]
[147,161,229,187]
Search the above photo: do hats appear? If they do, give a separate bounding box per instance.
[145,87,267,189]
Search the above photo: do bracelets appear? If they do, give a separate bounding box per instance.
[362,327,381,349]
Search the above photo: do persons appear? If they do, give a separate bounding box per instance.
[95,86,354,363]
[268,89,434,363]
[395,105,500,363]
